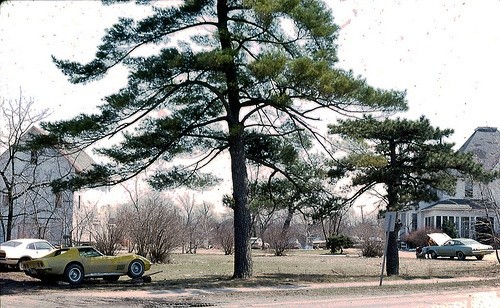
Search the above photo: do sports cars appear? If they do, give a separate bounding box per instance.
[23,245,152,286]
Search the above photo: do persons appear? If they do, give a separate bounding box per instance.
[428,238,437,259]
[416,246,426,259]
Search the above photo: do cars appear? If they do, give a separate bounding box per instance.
[422,233,494,261]
[250,237,262,249]
[0,238,68,270]
